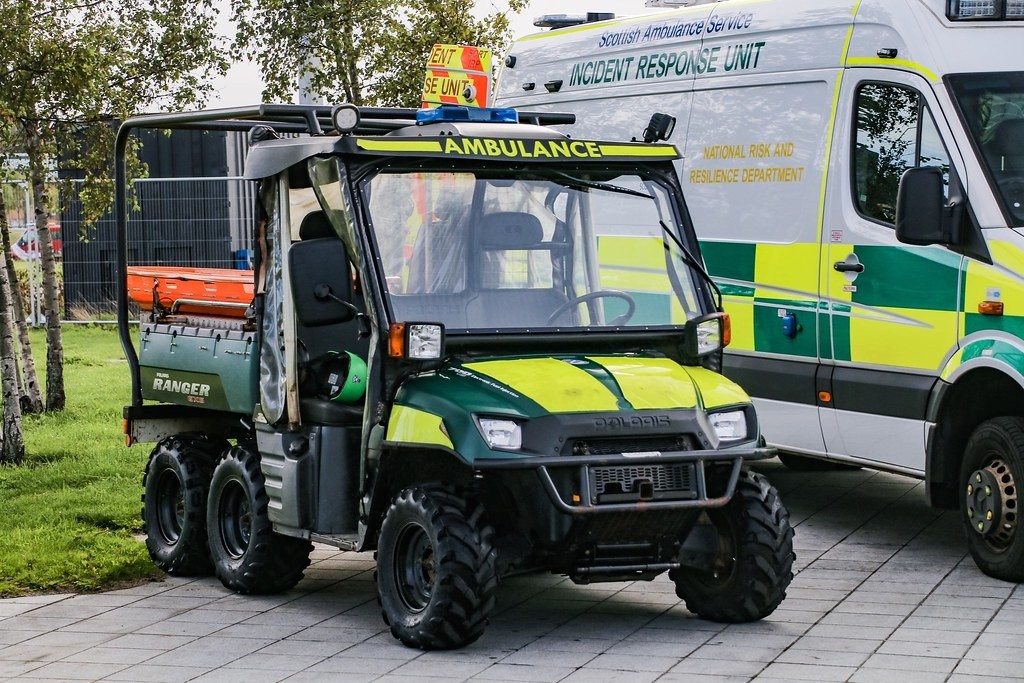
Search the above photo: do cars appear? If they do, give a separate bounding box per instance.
[10,224,63,260]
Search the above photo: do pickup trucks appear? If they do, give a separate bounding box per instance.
[113,37,803,649]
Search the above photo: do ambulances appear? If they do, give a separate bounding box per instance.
[486,1,1024,582]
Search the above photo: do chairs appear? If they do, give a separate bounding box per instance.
[288,212,573,428]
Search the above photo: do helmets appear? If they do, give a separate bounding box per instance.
[315,349,368,401]
